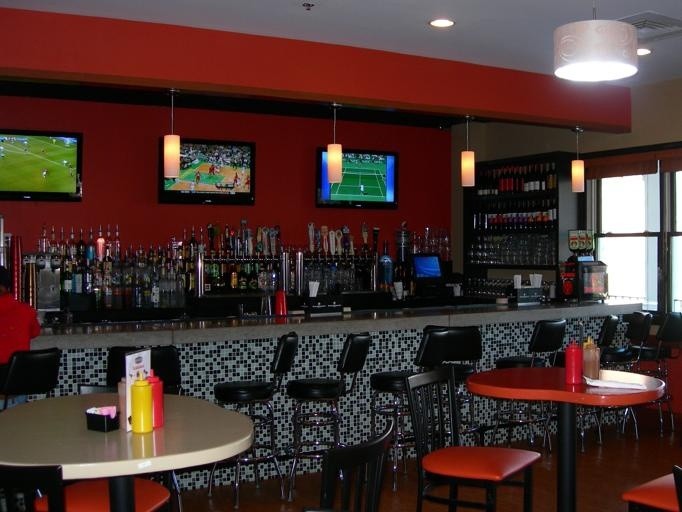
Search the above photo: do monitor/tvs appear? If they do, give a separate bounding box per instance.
[414,255,442,279]
[315,146,400,210]
[158,138,256,205]
[0,128,84,203]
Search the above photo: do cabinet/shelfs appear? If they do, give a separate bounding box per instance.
[461,150,582,303]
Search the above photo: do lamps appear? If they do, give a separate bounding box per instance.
[460,113,476,188]
[567,125,589,194]
[550,0,640,83]
[163,88,181,178]
[327,104,344,184]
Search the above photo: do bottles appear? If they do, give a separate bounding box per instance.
[275,290,289,318]
[567,228,595,256]
[199,217,287,297]
[117,377,128,432]
[146,368,165,431]
[379,221,413,303]
[466,276,554,302]
[289,247,375,299]
[581,335,594,349]
[12,222,202,316]
[473,160,558,200]
[129,371,155,435]
[565,339,582,385]
[305,219,381,257]
[471,197,559,232]
[410,225,453,264]
[583,340,600,382]
[465,230,557,267]
[261,296,272,318]
